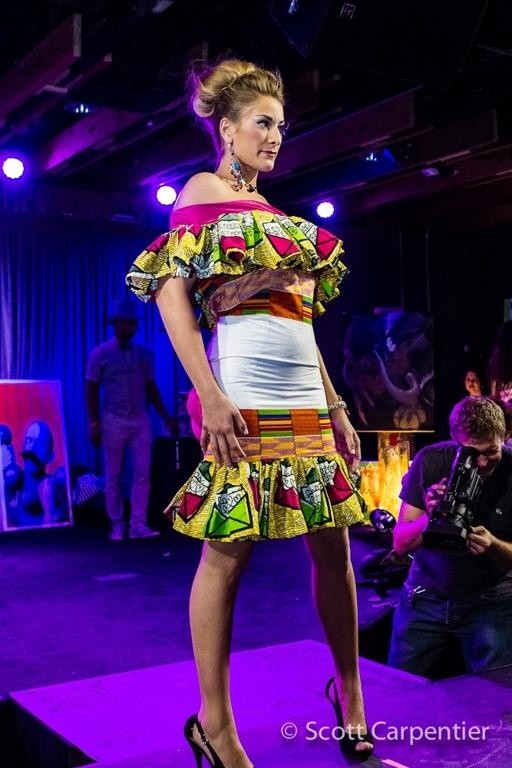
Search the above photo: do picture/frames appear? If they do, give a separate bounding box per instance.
[0,379,74,532]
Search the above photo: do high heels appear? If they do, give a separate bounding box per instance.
[322,675,378,765]
[181,710,262,768]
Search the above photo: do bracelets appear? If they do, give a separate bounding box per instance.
[326,401,348,413]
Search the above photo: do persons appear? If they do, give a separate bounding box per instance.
[84,297,182,541]
[330,329,511,684]
[1,421,68,528]
[124,61,378,767]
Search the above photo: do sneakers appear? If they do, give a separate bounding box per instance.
[109,523,163,542]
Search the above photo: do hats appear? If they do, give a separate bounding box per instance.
[103,297,148,324]
